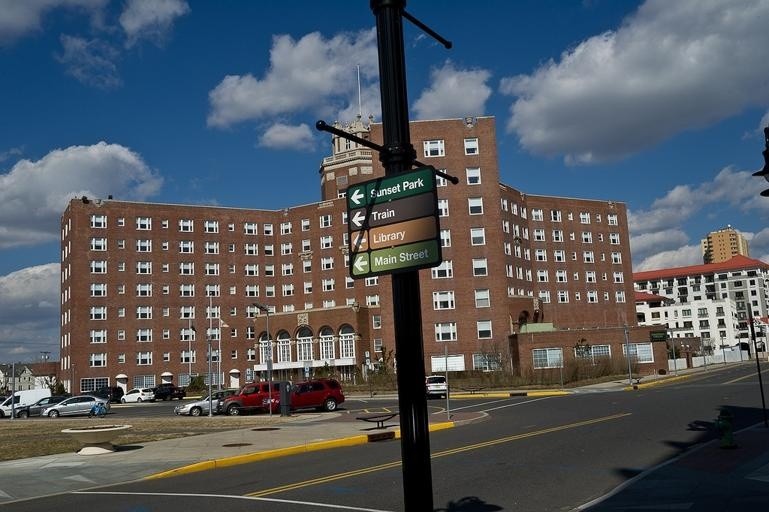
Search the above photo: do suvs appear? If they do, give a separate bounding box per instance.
[425,374,449,400]
[81,386,124,404]
[216,379,292,417]
[260,377,347,416]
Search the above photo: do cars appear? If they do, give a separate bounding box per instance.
[173,388,240,418]
[40,394,113,419]
[119,387,156,404]
[12,394,71,419]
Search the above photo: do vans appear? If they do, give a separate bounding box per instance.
[0,388,53,418]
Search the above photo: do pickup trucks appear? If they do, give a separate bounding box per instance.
[152,383,188,401]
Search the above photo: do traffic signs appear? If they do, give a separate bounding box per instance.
[348,191,435,231]
[351,239,438,276]
[348,169,434,208]
[350,215,436,253]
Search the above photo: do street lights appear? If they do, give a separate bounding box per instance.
[751,125,769,198]
[71,361,77,396]
[699,335,709,370]
[720,331,727,365]
[623,323,634,385]
[2,362,16,420]
[251,301,273,417]
[737,331,744,363]
[39,351,52,369]
[669,327,677,376]
[189,324,215,417]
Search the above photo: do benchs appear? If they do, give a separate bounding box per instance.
[355,412,399,428]
[459,386,486,394]
[632,376,644,384]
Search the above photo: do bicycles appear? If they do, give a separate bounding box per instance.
[87,402,108,419]
